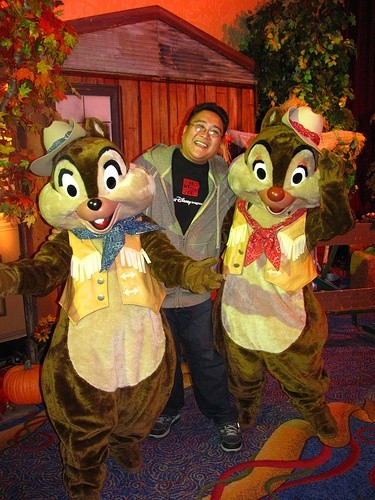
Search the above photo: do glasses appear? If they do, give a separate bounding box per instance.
[187,121,222,139]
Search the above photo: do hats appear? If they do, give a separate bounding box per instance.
[29,119,87,177]
[282,106,324,157]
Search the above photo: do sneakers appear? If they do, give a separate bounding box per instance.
[149,410,181,438]
[219,422,242,452]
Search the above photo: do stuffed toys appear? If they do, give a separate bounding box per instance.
[211,105,354,440]
[0,117,223,500]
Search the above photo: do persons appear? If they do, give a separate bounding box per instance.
[48,103,243,452]
[349,184,375,224]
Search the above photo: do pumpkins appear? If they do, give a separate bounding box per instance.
[2,360,43,405]
